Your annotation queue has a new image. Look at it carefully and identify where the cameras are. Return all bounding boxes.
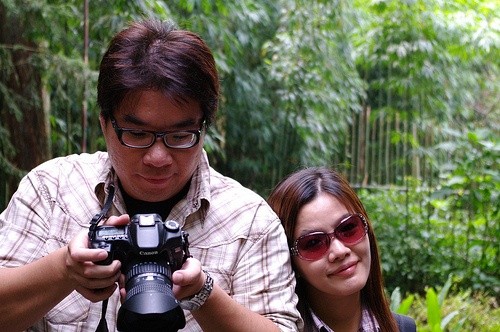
[91,214,190,332]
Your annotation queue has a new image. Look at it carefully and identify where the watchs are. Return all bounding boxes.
[174,269,214,313]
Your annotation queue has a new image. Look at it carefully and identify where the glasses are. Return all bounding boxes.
[108,112,206,149]
[291,213,368,262]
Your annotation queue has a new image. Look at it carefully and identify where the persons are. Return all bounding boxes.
[267,166,417,332]
[0,20,305,332]
